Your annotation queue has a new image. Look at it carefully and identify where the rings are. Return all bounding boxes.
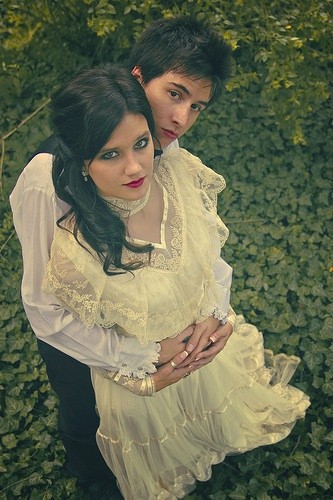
[169,360,178,369]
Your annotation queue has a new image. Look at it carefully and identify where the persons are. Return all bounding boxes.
[8,13,236,500]
[38,64,311,500]
